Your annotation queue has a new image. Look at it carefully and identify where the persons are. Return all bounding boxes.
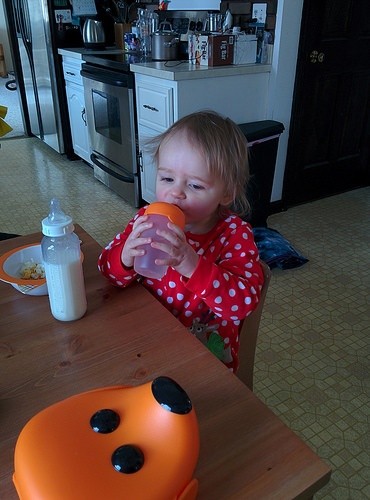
[98,110,264,374]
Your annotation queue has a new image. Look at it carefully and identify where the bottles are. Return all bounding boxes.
[135,201,186,277]
[41,199,87,321]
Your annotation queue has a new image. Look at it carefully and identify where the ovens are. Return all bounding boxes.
[81,64,144,208]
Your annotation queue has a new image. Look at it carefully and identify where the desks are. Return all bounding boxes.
[1,223,331,499]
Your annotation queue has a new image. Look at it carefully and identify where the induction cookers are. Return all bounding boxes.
[81,9,219,72]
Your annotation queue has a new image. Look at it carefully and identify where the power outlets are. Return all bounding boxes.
[252,3,266,23]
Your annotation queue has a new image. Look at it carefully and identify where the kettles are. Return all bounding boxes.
[80,16,106,50]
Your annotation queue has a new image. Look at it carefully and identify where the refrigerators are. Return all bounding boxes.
[10,0,118,160]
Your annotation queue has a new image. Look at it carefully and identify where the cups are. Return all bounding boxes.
[124,33,136,49]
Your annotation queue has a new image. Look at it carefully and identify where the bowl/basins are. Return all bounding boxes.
[0,242,83,295]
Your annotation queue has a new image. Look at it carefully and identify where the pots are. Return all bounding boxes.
[151,21,181,61]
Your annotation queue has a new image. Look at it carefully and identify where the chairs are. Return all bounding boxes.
[236,260,272,391]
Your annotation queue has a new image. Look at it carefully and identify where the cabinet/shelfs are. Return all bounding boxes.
[62,55,93,168]
[135,72,270,204]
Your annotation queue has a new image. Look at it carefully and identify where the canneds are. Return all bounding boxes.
[124,33,136,50]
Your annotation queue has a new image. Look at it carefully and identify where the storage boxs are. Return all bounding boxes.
[189,35,235,67]
